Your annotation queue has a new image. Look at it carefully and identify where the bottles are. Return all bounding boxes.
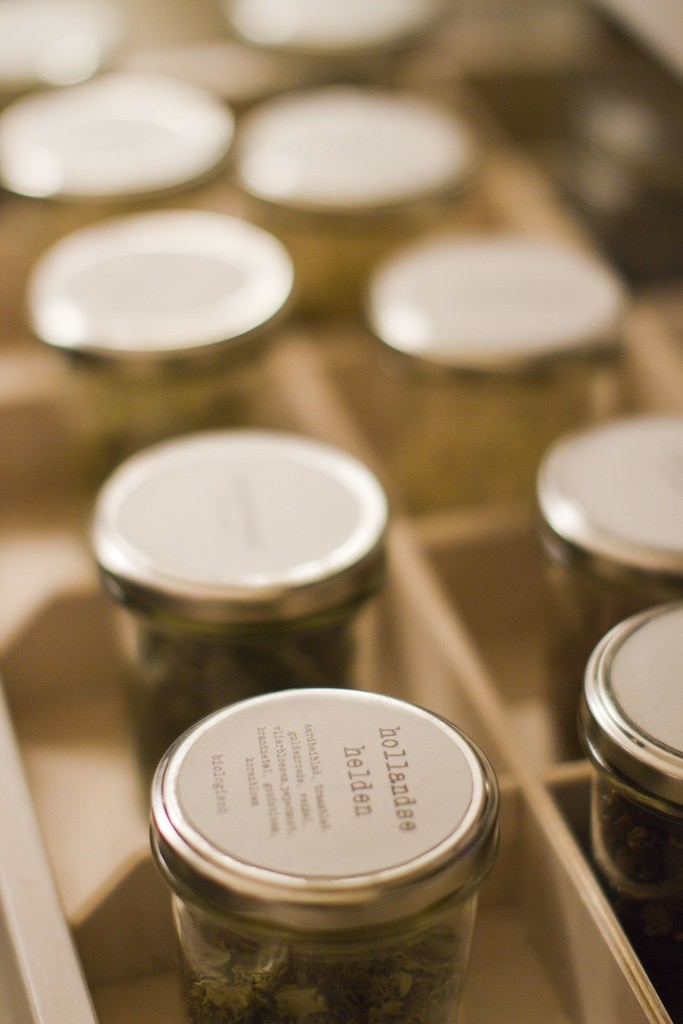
[1,0,683,1024]
